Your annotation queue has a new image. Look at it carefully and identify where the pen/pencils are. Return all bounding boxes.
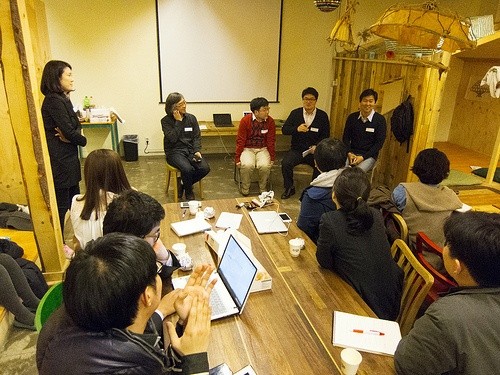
[182,209,186,217]
[353,328,385,336]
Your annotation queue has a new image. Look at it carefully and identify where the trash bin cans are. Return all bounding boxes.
[122,134,139,161]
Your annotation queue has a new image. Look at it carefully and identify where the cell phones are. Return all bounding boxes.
[352,158,356,162]
[279,212,292,223]
[194,155,202,162]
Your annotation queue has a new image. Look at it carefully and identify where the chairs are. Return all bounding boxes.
[367,147,383,190]
[388,213,460,338]
[162,131,205,203]
[234,154,273,194]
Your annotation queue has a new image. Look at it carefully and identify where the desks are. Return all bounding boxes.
[160,196,398,375]
[78,116,119,164]
[198,119,284,136]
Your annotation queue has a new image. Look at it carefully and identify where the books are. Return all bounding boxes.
[333,311,402,357]
[171,218,212,237]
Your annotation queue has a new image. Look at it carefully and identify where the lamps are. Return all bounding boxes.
[313,0,341,12]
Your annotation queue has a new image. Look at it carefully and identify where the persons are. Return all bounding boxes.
[342,89,387,173]
[394,210,500,375]
[0,253,40,331]
[391,148,463,271]
[281,87,330,199]
[161,92,210,201]
[296,137,348,246]
[35,231,217,375]
[71,149,138,250]
[102,191,183,334]
[236,97,275,196]
[40,60,87,262]
[316,167,405,321]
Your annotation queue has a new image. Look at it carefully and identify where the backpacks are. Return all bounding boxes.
[390,94,414,153]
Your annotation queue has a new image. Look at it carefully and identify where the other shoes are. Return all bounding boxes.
[63,244,75,259]
[177,177,185,199]
[242,188,249,196]
[21,301,41,312]
[260,189,267,194]
[14,316,37,330]
[186,187,195,201]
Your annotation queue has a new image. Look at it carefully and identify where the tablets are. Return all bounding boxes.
[180,202,201,208]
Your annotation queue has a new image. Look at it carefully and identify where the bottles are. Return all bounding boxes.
[83,96,95,109]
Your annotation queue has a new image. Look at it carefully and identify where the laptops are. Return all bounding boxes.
[213,114,234,127]
[249,210,288,235]
[168,233,258,323]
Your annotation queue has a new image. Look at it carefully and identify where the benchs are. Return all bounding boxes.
[0,204,42,347]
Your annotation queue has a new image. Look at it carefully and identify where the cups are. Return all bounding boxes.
[172,243,186,254]
[289,239,301,258]
[340,348,362,375]
[188,200,198,215]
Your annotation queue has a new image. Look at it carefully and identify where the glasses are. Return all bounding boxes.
[176,100,186,108]
[259,106,271,113]
[302,96,316,103]
[142,232,160,243]
[139,259,164,295]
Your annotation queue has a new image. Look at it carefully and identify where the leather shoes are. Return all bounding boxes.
[281,186,295,199]
